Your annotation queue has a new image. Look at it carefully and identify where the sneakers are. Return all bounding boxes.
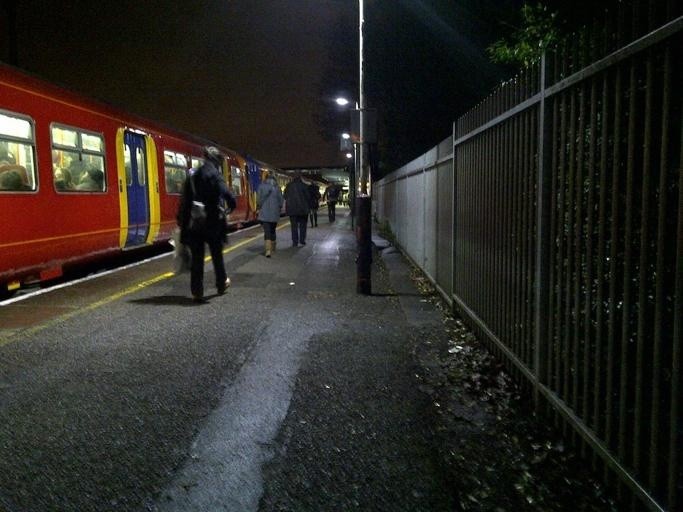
[218,277,230,294]
[193,290,205,302]
[292,240,306,247]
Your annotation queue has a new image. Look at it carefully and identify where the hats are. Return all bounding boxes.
[205,144,223,165]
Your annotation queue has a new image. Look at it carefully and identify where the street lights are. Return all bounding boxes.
[335,97,358,231]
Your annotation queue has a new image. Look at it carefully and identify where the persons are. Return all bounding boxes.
[283,176,311,246]
[307,181,321,227]
[256,174,283,258]
[0,143,104,192]
[176,145,236,303]
[323,183,338,223]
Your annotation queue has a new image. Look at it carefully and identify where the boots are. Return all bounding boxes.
[310,213,317,228]
[264,240,271,257]
[270,241,276,253]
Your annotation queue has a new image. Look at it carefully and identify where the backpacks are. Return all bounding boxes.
[329,187,337,198]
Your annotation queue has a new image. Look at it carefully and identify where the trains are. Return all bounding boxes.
[0,63,328,301]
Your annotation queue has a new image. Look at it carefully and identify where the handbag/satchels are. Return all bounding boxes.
[190,202,225,233]
